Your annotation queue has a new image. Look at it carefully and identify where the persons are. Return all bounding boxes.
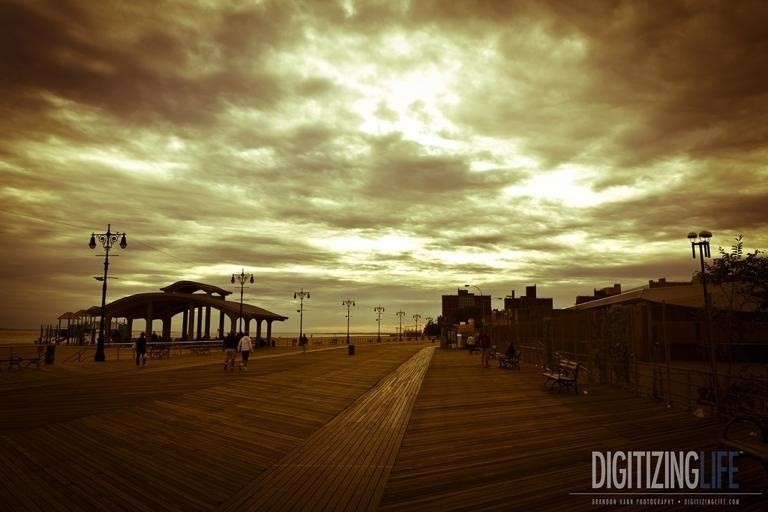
[504,342,517,368]
[151,331,158,342]
[478,328,493,369]
[135,332,147,367]
[302,333,308,344]
[223,328,253,372]
[466,334,476,355]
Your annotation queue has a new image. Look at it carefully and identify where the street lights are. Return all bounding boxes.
[341,299,433,343]
[88,223,127,362]
[464,284,486,325]
[293,287,310,346]
[687,230,723,423]
[231,268,254,332]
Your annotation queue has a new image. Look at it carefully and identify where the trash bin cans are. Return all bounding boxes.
[45,346,55,363]
[457,336,463,348]
[255,340,260,348]
[432,339,435,343]
[272,341,275,347]
[440,336,448,349]
[349,346,355,356]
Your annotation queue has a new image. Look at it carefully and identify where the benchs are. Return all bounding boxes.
[191,346,211,355]
[719,417,767,474]
[541,359,582,394]
[8,355,40,371]
[149,347,169,359]
[503,351,521,369]
